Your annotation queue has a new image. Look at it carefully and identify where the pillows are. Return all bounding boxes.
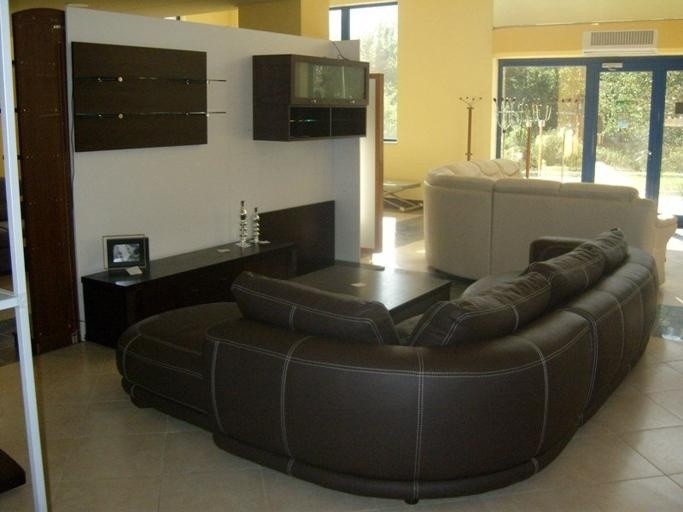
[408,272,552,346]
[230,269,402,346]
[573,227,630,271]
[519,247,606,312]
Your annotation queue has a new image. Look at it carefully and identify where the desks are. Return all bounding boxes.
[383,179,423,213]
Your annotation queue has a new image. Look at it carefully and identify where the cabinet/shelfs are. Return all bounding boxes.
[252,54,370,141]
[81,239,296,350]
[71,41,227,153]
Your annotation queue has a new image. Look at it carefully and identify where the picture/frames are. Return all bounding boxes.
[103,234,150,276]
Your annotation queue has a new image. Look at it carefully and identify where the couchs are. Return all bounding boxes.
[117,235,659,505]
[421,159,678,286]
